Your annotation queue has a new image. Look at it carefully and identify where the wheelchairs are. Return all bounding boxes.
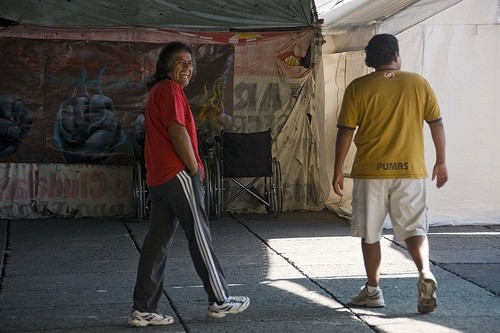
[214,128,283,221]
[133,133,213,223]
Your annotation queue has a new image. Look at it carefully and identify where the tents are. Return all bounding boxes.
[0,0,500,230]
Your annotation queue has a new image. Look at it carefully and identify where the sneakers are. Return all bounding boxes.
[127,310,175,327]
[351,282,386,308]
[417,272,438,313]
[207,295,250,318]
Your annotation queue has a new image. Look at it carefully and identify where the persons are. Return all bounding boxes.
[332,32,449,313]
[0,95,33,162]
[128,42,251,326]
[54,95,121,165]
